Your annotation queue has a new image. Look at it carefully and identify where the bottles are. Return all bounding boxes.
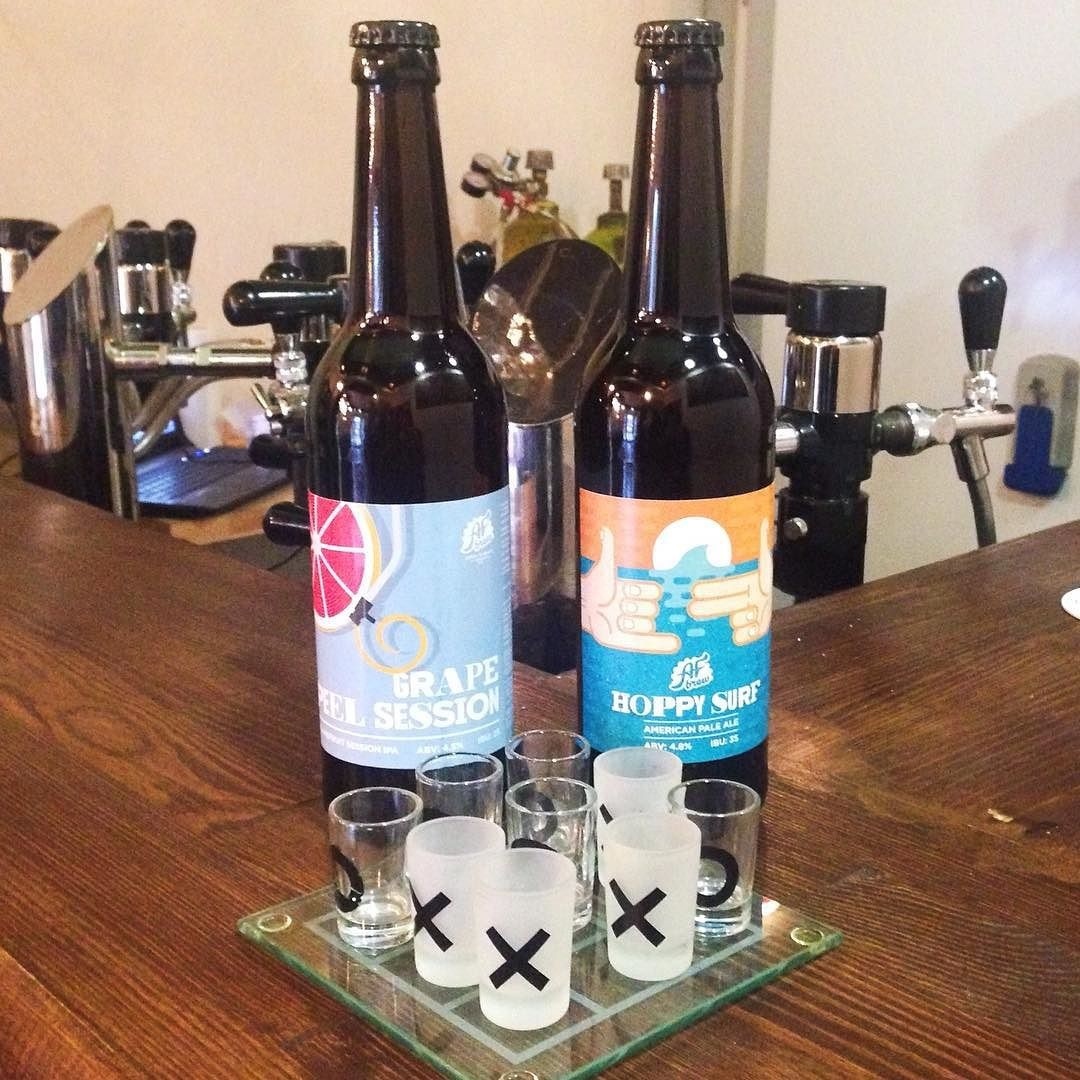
[298,18,517,812]
[568,14,781,827]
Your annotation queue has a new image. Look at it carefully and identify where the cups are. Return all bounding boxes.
[327,725,760,1030]
[504,413,573,606]
